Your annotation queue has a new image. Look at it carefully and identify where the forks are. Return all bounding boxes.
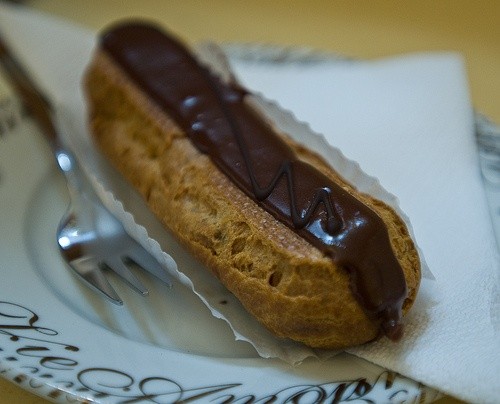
[0,30,175,306]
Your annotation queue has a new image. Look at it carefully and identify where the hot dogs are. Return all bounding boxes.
[80,16,421,350]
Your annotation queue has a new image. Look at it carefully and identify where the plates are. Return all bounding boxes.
[0,45,498,404]
